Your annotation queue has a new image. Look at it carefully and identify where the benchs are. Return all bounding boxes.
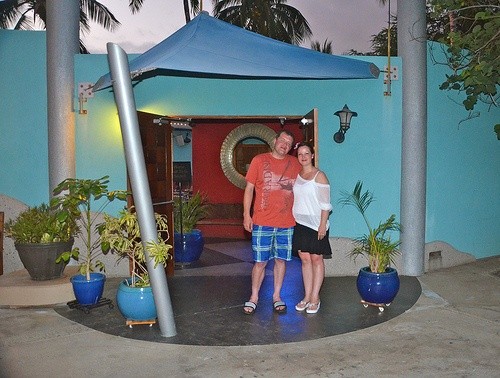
[193,203,253,239]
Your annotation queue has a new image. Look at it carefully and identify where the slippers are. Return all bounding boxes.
[273,301,286,312]
[306,301,320,314]
[295,301,310,311]
[243,302,256,314]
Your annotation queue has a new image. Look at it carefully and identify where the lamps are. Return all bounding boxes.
[334,104,358,142]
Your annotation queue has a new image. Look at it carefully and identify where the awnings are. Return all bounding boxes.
[91,10,381,93]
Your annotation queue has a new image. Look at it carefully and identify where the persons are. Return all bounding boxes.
[243,130,302,315]
[292,143,332,314]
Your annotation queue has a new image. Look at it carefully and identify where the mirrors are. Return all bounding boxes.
[220,123,278,190]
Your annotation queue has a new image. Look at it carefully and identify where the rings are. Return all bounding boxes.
[322,234,324,236]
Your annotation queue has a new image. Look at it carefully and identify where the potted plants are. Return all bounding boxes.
[173,190,211,263]
[339,179,404,303]
[51,176,132,305]
[103,205,173,320]
[2,202,81,281]
